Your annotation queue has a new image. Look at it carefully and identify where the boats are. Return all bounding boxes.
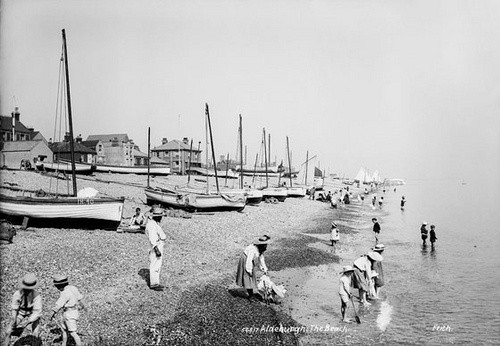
[96,164,174,177]
[36,154,98,176]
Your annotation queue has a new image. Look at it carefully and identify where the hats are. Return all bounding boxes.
[339,265,357,274]
[254,234,272,244]
[369,252,383,261]
[373,244,386,252]
[332,222,337,227]
[152,207,164,217]
[18,275,39,289]
[52,275,69,284]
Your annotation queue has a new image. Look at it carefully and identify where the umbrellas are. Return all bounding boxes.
[75,187,99,201]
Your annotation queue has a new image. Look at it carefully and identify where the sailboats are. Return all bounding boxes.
[1,28,126,231]
[353,164,386,187]
[224,126,288,203]
[185,141,240,180]
[251,136,306,199]
[285,167,301,178]
[235,132,286,178]
[184,113,263,205]
[143,102,248,215]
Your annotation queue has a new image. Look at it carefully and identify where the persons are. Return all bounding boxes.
[140,204,159,228]
[49,276,88,346]
[5,273,42,346]
[360,244,386,300]
[128,208,144,226]
[145,208,165,291]
[421,221,428,248]
[339,265,358,322]
[305,185,350,209]
[330,222,340,246]
[365,252,383,300]
[372,218,380,244]
[379,197,384,208]
[351,257,371,307]
[401,197,406,210]
[236,235,273,302]
[372,197,377,206]
[430,225,437,252]
